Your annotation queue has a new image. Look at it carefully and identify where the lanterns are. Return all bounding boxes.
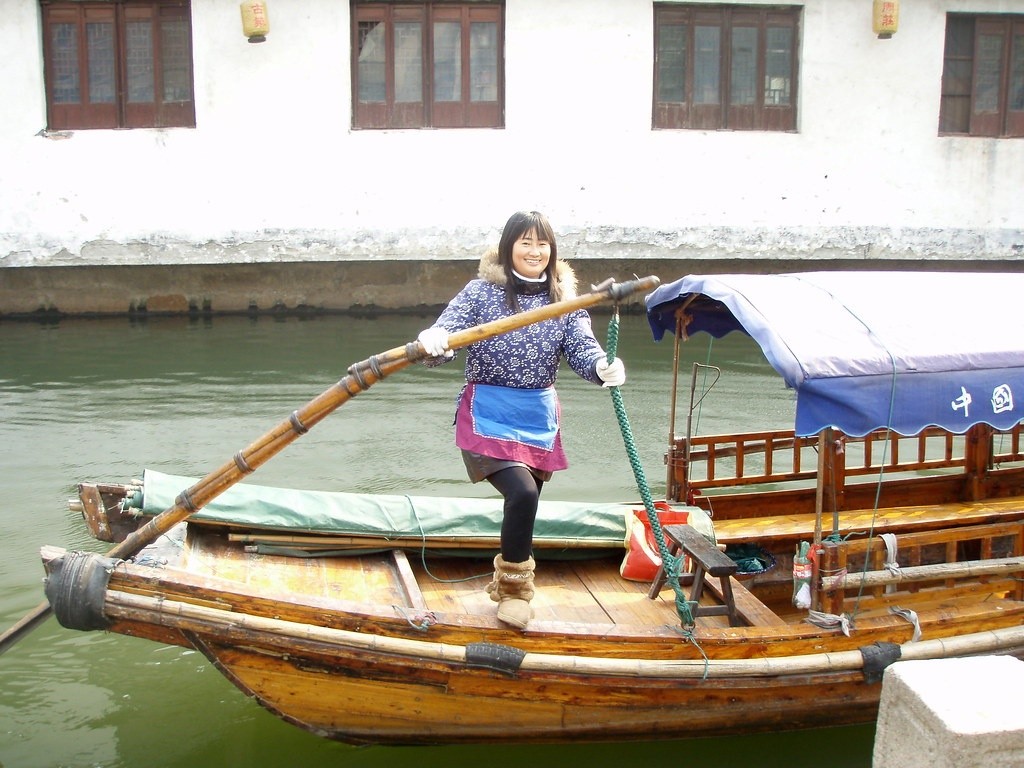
[240,0,270,43]
[873,0,900,39]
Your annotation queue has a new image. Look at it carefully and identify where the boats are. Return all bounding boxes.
[33,273,1024,748]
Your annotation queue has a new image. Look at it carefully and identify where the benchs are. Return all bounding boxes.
[712,496,1024,544]
[648,524,737,626]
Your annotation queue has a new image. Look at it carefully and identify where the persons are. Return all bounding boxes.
[418,211,626,628]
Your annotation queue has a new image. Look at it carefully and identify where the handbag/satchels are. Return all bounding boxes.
[619,501,693,581]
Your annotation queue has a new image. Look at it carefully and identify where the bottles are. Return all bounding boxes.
[791,555,813,609]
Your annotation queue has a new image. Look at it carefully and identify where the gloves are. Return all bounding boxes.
[418,327,456,359]
[595,357,625,387]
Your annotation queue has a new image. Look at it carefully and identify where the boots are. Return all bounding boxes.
[484,554,537,630]
